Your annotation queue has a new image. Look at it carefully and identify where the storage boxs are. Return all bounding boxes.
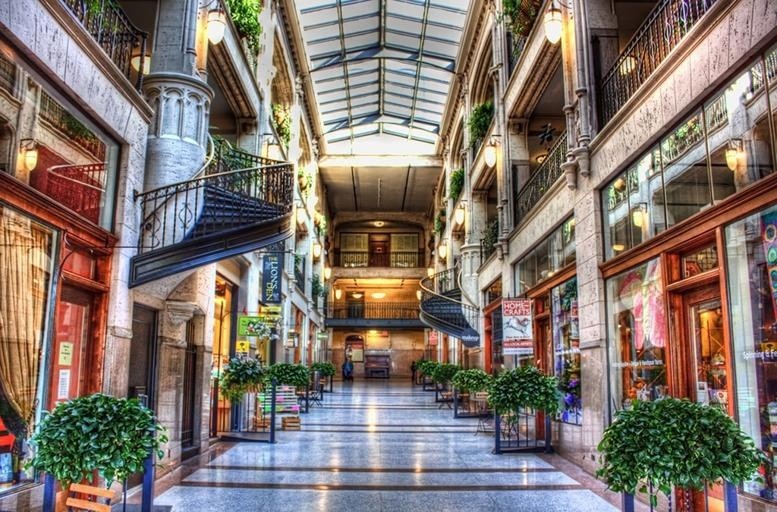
[254,418,272,433]
[281,416,301,431]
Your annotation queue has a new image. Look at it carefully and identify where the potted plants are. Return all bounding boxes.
[212,353,273,406]
[297,167,314,192]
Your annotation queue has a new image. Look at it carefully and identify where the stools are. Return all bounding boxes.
[437,391,499,437]
[295,389,321,414]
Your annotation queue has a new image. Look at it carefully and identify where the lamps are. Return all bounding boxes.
[544,0,572,46]
[201,1,227,46]
[633,202,647,228]
[294,206,344,302]
[411,134,503,302]
[19,137,40,172]
[724,137,744,171]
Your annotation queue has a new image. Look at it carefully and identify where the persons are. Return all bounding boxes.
[342,356,354,383]
[411,361,416,383]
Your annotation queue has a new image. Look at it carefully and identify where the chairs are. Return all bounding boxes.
[65,482,116,512]
